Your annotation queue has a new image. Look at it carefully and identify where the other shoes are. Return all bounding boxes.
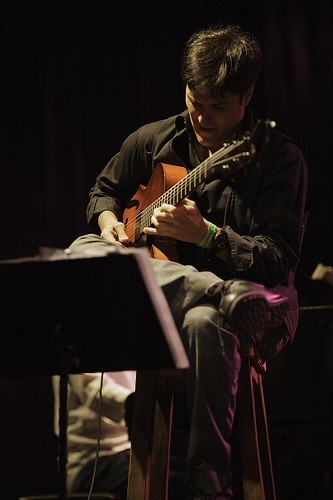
[215,279,288,331]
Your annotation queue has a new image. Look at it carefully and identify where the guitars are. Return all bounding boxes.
[118,119,277,264]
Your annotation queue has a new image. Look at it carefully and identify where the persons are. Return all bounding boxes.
[38,25,307,500]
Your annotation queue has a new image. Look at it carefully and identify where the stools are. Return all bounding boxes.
[127,343,277,500]
